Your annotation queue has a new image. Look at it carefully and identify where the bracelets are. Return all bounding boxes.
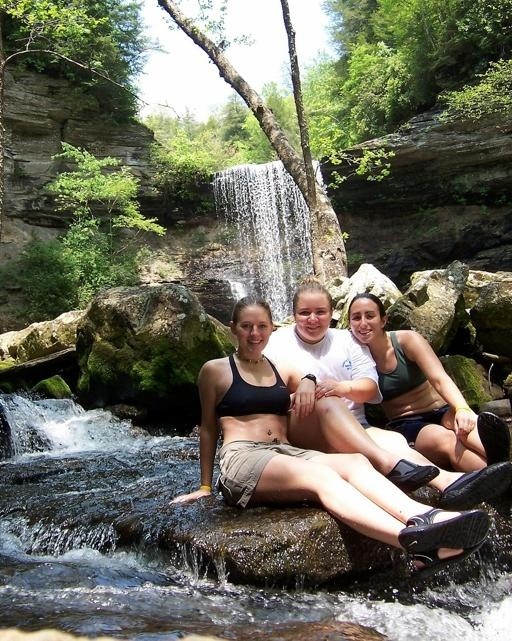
[453,403,473,415]
[199,485,213,493]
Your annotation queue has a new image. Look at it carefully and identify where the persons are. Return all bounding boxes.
[167,294,492,580]
[348,293,512,521]
[258,277,512,509]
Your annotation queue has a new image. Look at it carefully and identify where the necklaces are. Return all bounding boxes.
[235,349,267,365]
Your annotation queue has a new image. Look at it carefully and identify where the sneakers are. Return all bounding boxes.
[441,461,512,509]
[384,460,439,494]
[478,412,510,466]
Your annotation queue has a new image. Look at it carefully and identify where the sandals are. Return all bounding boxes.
[408,541,486,580]
[398,509,490,552]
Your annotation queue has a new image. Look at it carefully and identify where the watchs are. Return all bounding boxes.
[300,374,317,387]
